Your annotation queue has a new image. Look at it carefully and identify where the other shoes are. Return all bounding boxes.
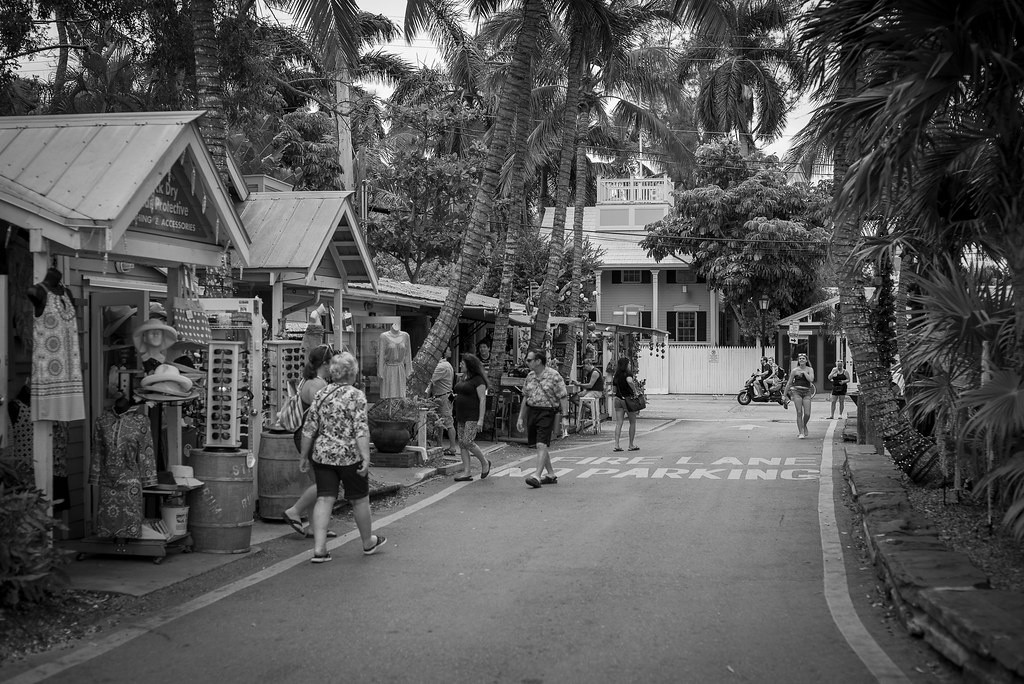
[481,461,491,479]
[799,427,808,438]
[455,475,473,481]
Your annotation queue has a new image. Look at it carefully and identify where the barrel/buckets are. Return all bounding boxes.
[161,506,189,535]
[189,448,254,554]
[258,431,316,519]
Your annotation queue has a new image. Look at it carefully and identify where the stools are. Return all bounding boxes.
[576,397,600,436]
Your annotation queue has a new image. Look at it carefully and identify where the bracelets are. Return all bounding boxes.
[562,415,568,419]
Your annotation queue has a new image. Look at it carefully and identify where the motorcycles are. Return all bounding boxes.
[737,368,791,405]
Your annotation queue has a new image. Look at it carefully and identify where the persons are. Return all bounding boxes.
[826,362,850,419]
[475,338,491,374]
[442,346,451,361]
[8,375,72,511]
[763,357,782,395]
[612,358,640,451]
[281,345,337,537]
[784,354,814,438]
[300,304,326,350]
[517,348,569,488]
[376,322,412,399]
[297,351,387,562]
[87,397,159,538]
[570,358,604,417]
[431,356,455,456]
[454,353,492,482]
[754,356,772,398]
[141,329,165,376]
[26,268,86,421]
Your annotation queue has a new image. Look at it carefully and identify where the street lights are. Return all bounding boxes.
[759,291,771,373]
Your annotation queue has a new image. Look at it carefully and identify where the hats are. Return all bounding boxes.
[171,464,205,487]
[103,306,138,338]
[133,318,177,353]
[133,356,205,401]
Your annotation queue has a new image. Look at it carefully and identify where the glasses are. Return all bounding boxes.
[318,345,329,361]
[798,353,806,357]
[211,347,305,440]
[527,358,536,362]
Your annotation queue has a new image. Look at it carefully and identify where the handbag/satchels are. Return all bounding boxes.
[173,264,212,348]
[625,389,646,412]
[552,412,561,436]
[783,398,788,409]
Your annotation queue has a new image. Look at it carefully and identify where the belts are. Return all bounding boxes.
[435,390,453,398]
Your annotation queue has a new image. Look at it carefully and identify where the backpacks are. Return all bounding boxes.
[778,367,785,379]
[280,375,326,433]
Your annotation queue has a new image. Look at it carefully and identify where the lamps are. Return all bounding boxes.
[115,261,134,273]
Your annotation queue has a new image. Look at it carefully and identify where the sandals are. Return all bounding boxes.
[526,477,541,488]
[541,476,557,483]
[443,448,456,455]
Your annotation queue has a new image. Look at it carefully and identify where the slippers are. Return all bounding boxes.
[311,552,332,562]
[613,447,624,451]
[630,445,639,450]
[363,535,388,555]
[305,530,335,538]
[281,510,305,536]
